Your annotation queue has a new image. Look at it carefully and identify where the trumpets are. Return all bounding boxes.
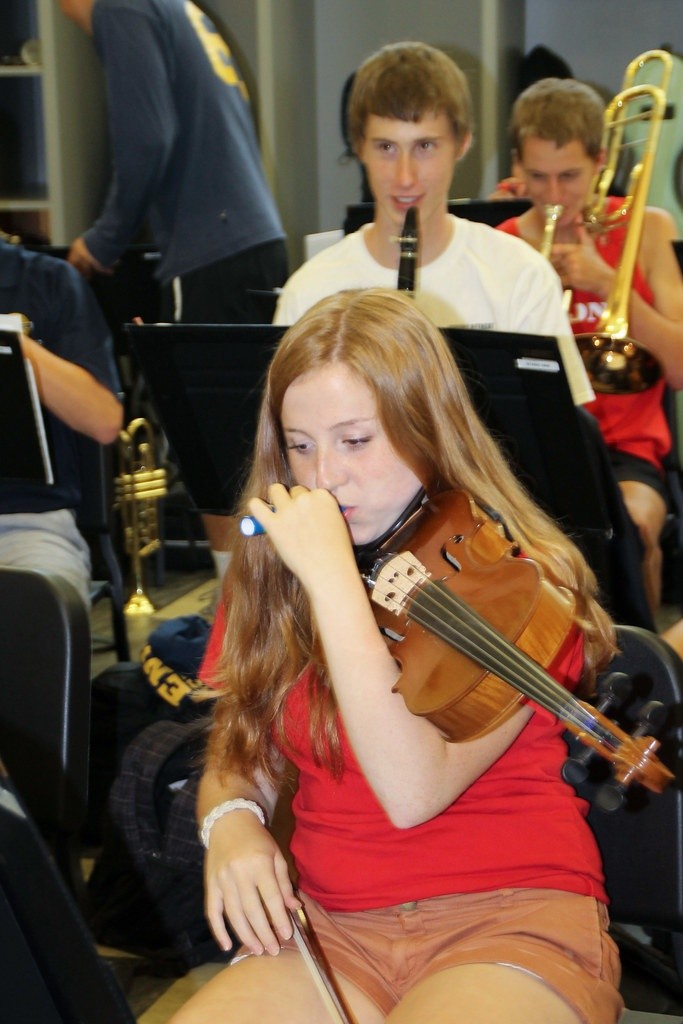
[115,418,167,616]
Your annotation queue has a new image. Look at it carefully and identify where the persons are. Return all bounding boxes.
[0,241,124,612]
[274,39,683,674]
[56,0,288,583]
[166,288,622,1024]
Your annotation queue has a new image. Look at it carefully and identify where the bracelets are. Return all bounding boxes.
[200,797,265,849]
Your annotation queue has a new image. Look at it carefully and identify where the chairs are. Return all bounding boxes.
[0,434,131,1024]
[561,625,683,1024]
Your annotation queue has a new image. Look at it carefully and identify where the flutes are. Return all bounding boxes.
[393,208,419,292]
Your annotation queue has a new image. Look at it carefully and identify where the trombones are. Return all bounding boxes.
[540,50,675,395]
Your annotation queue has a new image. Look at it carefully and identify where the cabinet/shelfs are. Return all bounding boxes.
[1,0,523,279]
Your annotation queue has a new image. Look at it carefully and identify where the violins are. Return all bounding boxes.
[362,485,676,810]
